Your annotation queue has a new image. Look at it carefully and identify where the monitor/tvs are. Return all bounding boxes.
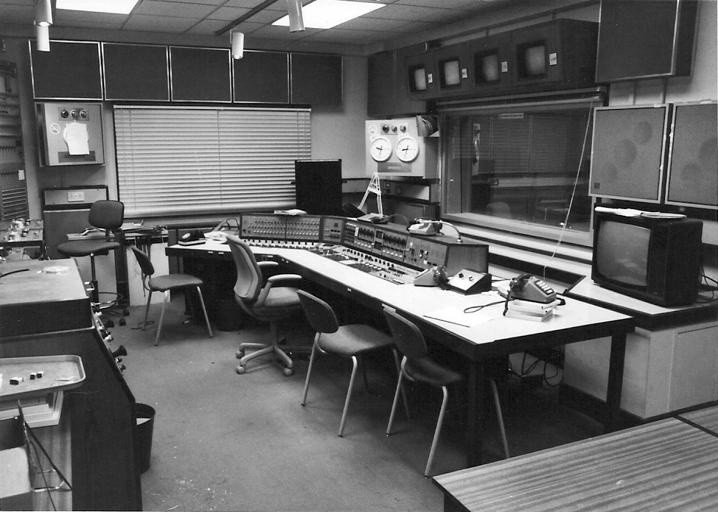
[591,210,703,308]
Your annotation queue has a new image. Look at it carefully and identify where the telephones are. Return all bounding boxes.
[509,272,557,303]
[413,264,450,287]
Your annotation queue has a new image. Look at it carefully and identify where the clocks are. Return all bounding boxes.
[368,137,392,162]
[394,137,420,163]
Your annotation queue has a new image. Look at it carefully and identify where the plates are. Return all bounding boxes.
[44,265,69,273]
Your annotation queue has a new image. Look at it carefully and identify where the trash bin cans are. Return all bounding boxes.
[136,403,156,473]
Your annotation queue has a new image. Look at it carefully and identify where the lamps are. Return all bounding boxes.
[36,0,54,27]
[231,32,244,61]
[287,0,304,33]
[36,21,51,53]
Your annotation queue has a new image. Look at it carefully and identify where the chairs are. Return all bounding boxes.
[131,246,214,346]
[296,288,413,437]
[56,199,130,329]
[225,234,316,377]
[379,304,510,477]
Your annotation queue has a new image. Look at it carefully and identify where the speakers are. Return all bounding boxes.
[588,104,667,205]
[664,100,716,210]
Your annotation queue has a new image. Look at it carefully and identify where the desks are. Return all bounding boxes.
[165,240,302,315]
[277,250,633,468]
[678,406,716,436]
[431,417,718,512]
[66,232,167,307]
